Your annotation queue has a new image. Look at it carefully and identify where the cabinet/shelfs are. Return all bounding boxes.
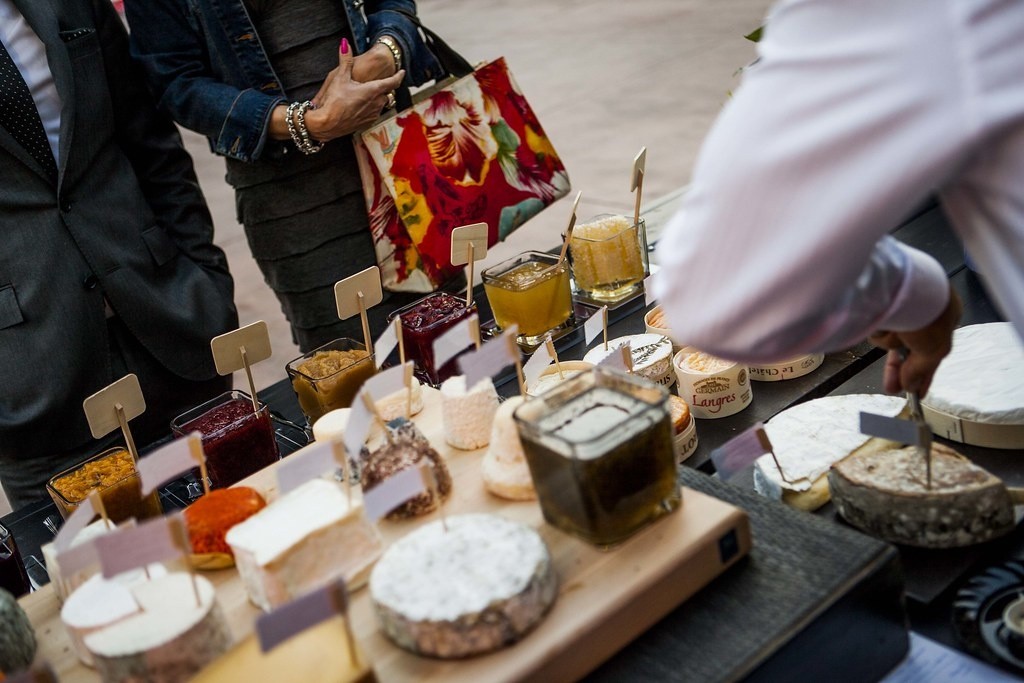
[0,188,1024,683]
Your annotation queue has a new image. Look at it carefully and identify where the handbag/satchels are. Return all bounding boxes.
[353,7,571,293]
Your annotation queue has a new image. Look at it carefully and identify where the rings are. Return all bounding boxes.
[386,92,397,109]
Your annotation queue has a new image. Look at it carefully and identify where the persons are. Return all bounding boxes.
[650,1,1024,395]
[0,0,238,511]
[122,0,452,365]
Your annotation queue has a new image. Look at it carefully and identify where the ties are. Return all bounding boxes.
[0,39,58,178]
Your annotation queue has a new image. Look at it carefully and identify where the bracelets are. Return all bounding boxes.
[284,101,325,156]
[377,37,401,71]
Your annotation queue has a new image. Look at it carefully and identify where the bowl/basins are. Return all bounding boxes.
[673,346,754,419]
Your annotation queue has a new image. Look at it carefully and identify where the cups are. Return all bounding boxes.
[45,446,142,527]
[386,291,479,390]
[285,337,376,428]
[480,250,576,345]
[513,364,682,550]
[169,390,280,493]
[561,215,651,304]
